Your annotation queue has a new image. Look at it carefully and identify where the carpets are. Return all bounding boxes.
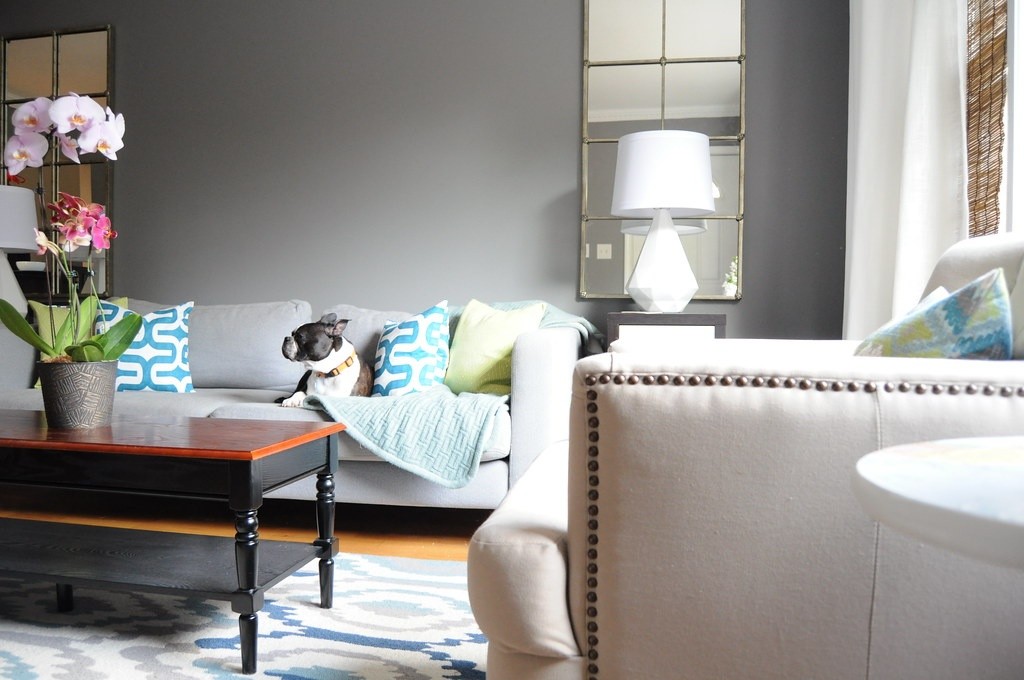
[1,551,489,680]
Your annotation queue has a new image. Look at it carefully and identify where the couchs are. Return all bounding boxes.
[466,231,1024,680]
[0,299,583,510]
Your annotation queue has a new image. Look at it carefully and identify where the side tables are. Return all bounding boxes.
[607,312,727,350]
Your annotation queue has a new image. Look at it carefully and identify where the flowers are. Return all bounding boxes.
[722,256,738,296]
[3,95,143,364]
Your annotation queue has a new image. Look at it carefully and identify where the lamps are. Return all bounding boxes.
[611,130,714,313]
[0,185,40,319]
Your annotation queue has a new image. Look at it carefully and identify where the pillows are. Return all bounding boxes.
[371,299,451,397]
[28,301,129,387]
[94,299,195,392]
[854,266,1013,360]
[445,299,547,396]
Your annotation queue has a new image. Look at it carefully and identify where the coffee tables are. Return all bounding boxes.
[0,409,347,673]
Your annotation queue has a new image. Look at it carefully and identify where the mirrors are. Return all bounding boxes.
[578,0,744,302]
[0,22,118,295]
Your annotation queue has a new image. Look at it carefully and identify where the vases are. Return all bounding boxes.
[36,361,117,430]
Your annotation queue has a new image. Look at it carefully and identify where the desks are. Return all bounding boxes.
[857,433,1023,571]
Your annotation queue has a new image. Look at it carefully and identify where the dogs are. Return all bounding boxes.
[273,313,374,408]
